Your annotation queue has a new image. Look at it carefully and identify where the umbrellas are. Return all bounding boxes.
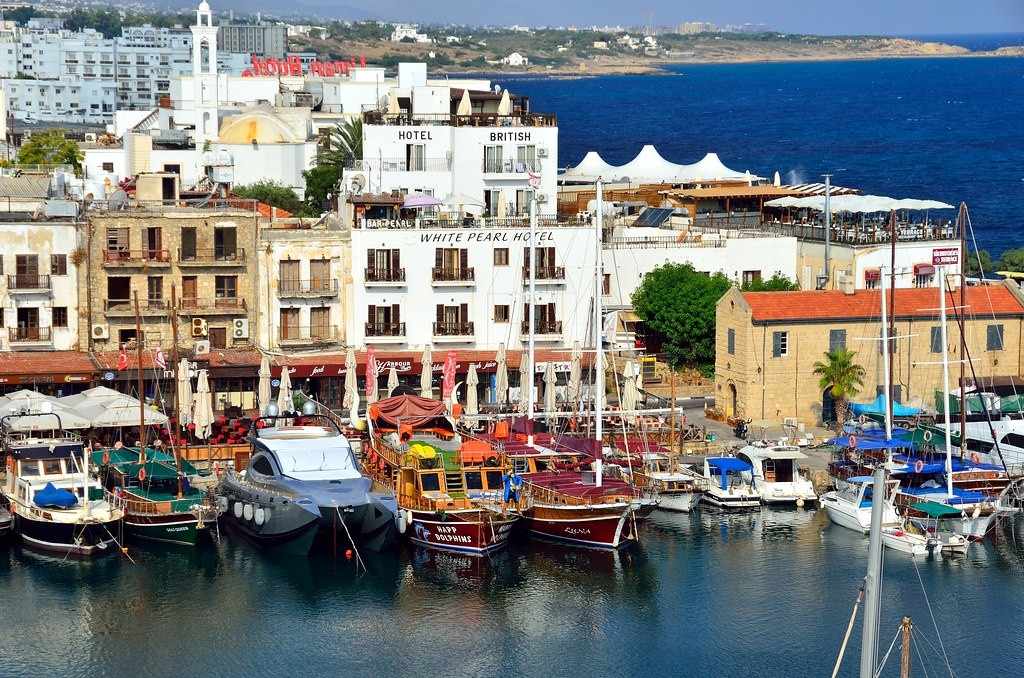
[542,362,556,433]
[277,366,294,427]
[178,358,193,446]
[193,370,215,445]
[495,342,508,410]
[594,351,636,432]
[696,166,780,187]
[568,340,583,403]
[403,191,485,218]
[421,345,478,433]
[258,358,271,424]
[764,193,955,234]
[342,349,400,412]
[520,352,529,412]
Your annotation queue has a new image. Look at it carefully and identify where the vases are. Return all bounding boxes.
[707,414,724,422]
[727,421,736,427]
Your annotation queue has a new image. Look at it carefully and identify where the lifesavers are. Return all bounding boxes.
[368,448,372,458]
[373,453,377,462]
[139,468,146,481]
[364,444,368,452]
[102,452,109,464]
[379,459,384,469]
[6,455,12,466]
[10,502,15,515]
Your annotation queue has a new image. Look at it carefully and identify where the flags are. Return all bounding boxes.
[117,348,128,371]
[156,344,166,371]
[528,173,541,188]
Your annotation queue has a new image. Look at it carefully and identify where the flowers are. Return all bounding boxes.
[704,409,724,417]
[726,416,745,424]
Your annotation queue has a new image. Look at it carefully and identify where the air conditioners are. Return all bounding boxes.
[194,340,210,354]
[233,319,249,338]
[783,418,797,429]
[192,318,207,336]
[537,147,549,157]
[537,193,548,202]
[91,323,109,339]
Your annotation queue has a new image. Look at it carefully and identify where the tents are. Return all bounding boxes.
[849,394,924,418]
[573,145,761,184]
[0,386,177,459]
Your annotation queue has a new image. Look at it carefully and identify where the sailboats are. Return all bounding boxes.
[817,201,1024,556]
[472,177,662,549]
[87,285,222,547]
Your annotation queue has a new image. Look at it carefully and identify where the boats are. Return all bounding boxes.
[0,378,127,562]
[641,365,818,512]
[216,413,398,557]
[358,392,534,557]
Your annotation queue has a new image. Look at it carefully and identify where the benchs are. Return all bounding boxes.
[374,426,455,441]
